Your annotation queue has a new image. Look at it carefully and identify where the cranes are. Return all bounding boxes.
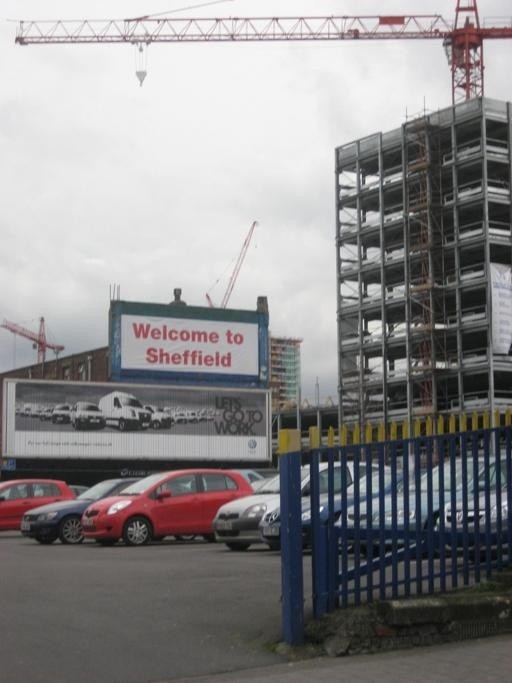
[204,220,258,312]
[8,0,512,105]
[0,313,65,363]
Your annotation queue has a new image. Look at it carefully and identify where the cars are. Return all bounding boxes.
[17,390,217,431]
